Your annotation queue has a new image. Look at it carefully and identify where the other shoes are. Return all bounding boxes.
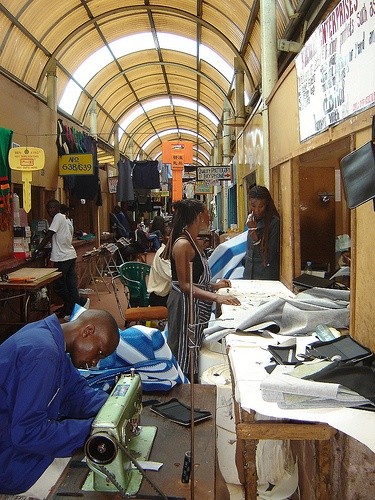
[80,297,90,309]
[57,310,72,318]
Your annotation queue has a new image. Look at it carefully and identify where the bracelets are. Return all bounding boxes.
[34,249,39,255]
[248,228,258,237]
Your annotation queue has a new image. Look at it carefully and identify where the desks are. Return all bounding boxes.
[216,279,336,500]
[0,268,62,330]
[46,384,216,500]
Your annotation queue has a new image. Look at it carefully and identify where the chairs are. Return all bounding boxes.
[111,274,168,329]
[120,261,151,320]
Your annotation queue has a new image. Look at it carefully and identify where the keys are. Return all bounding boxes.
[161,139,193,203]
[8,146,45,214]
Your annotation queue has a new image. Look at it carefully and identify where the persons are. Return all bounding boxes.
[27,200,88,320]
[244,185,281,280]
[166,197,241,385]
[113,205,172,307]
[0,309,140,500]
